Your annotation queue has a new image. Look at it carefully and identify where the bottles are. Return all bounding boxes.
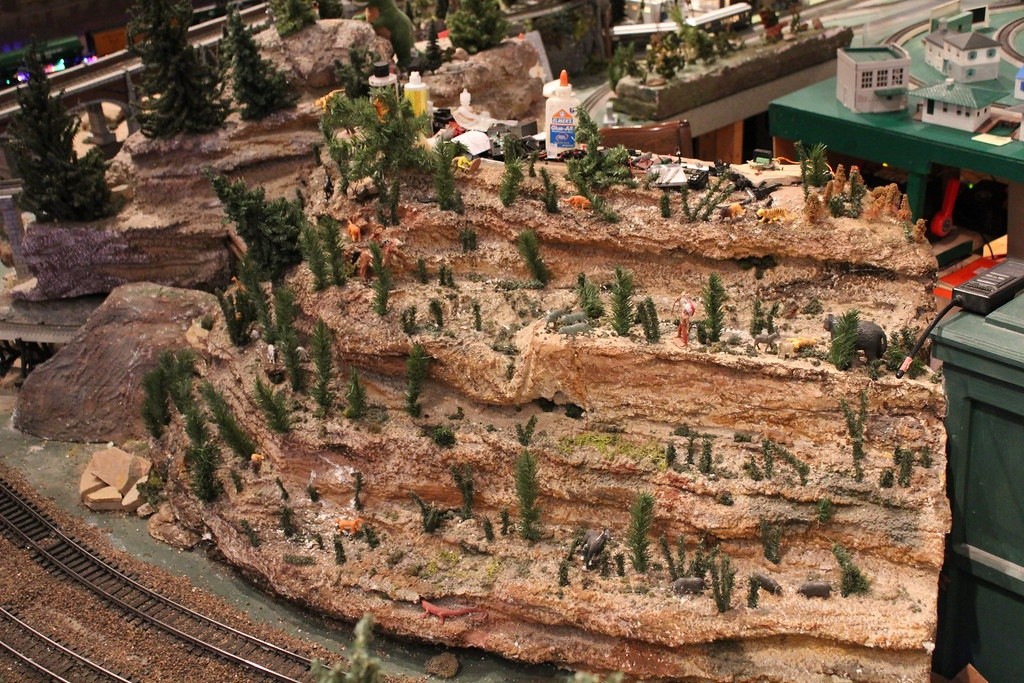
[456,88,476,125]
[546,72,581,159]
[367,61,399,124]
[404,70,429,117]
[432,109,455,135]
